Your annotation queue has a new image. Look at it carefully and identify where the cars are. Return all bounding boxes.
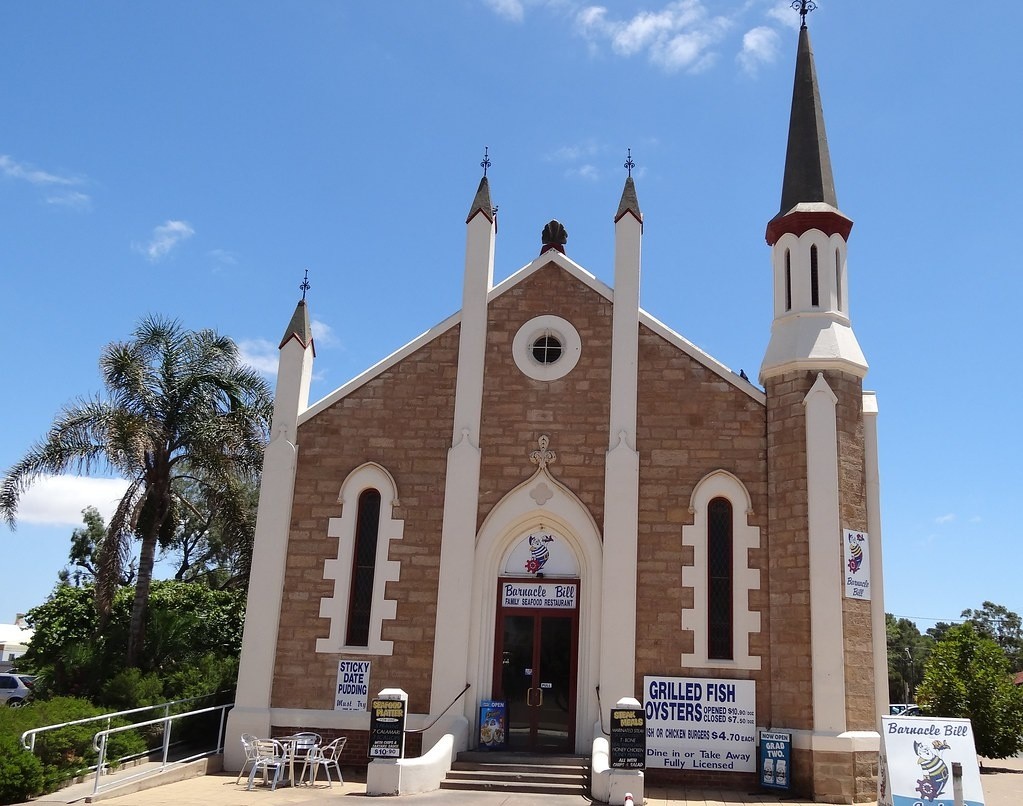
[891,704,934,718]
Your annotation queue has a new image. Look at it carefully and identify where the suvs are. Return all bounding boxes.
[0,671,40,708]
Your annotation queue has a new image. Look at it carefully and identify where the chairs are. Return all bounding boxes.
[298,736,347,790]
[236,732,284,787]
[282,732,323,788]
[245,739,290,792]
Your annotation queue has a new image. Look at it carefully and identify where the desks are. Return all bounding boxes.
[272,737,319,788]
